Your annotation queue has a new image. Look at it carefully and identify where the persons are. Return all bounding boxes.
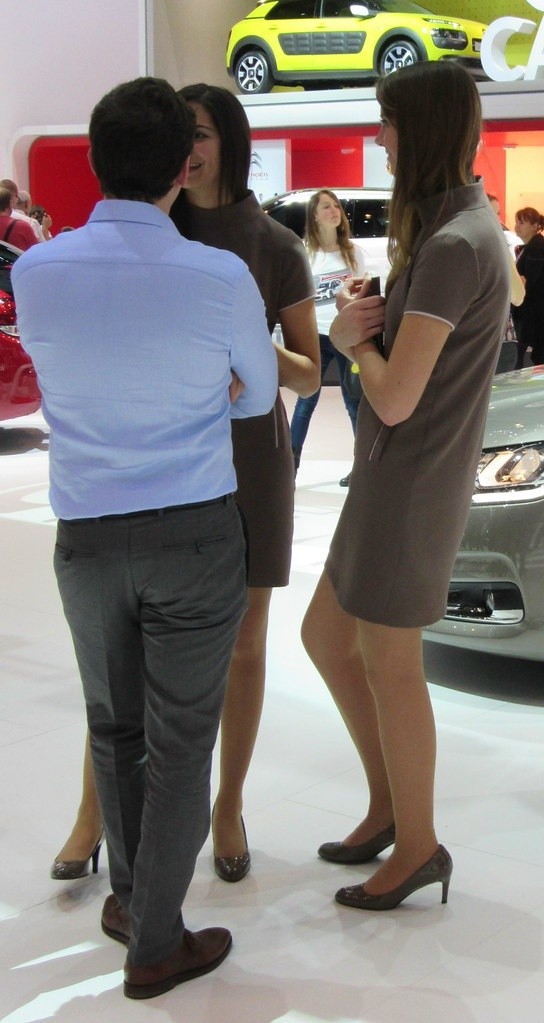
[290,189,366,492]
[486,193,544,375]
[1,76,322,997]
[300,60,512,910]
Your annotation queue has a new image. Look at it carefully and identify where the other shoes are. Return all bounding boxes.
[339,471,352,486]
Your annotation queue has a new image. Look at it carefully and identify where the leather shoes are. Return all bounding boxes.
[101,893,132,945]
[123,927,232,999]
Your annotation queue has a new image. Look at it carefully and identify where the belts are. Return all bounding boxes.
[99,491,235,520]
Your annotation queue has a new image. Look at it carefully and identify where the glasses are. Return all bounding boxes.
[15,194,20,205]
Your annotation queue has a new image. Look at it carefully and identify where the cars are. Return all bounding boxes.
[417,364,544,660]
[227,0,492,94]
[264,186,522,299]
[0,241,42,423]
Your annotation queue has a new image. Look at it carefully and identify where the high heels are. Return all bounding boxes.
[317,822,395,863]
[50,828,106,879]
[334,844,453,911]
[212,803,251,882]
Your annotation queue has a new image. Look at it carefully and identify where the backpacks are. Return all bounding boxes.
[0,240,25,298]
